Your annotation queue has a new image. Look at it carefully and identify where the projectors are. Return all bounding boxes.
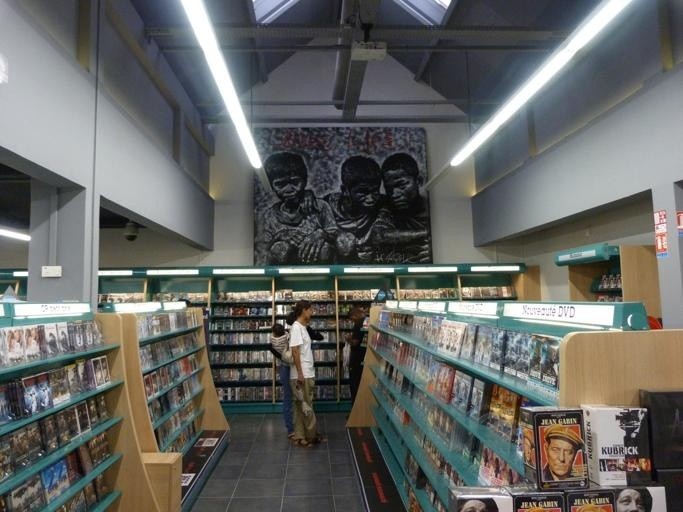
[351,39,387,62]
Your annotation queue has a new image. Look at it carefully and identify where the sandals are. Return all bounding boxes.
[293,437,312,448]
[288,431,294,439]
[308,436,329,447]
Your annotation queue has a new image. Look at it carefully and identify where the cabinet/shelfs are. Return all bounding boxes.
[554,244,661,319]
[208,275,274,413]
[273,275,337,414]
[345,302,683,512]
[102,301,231,512]
[144,278,209,328]
[97,279,146,304]
[337,273,399,413]
[460,274,523,301]
[0,279,21,294]
[0,302,161,512]
[398,274,462,300]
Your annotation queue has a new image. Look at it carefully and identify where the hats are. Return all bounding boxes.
[522,428,535,447]
[545,424,587,451]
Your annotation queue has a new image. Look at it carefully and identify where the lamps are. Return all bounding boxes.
[417,0,636,199]
[0,228,32,243]
[179,0,272,194]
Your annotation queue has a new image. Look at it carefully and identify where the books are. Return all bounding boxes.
[314,366,337,379]
[94,473,113,500]
[148,371,204,425]
[341,366,349,379]
[88,397,99,423]
[462,286,515,298]
[26,421,44,461]
[164,407,206,455]
[484,385,520,441]
[505,485,565,512]
[0,371,54,427]
[313,349,336,362]
[66,452,79,481]
[96,395,113,420]
[46,368,69,402]
[340,303,353,312]
[135,309,199,342]
[362,334,368,345]
[314,385,336,400]
[406,455,426,490]
[362,317,370,328]
[340,331,353,342]
[517,397,546,458]
[66,489,88,512]
[225,291,272,302]
[68,319,84,352]
[400,288,458,299]
[55,504,67,512]
[138,330,203,373]
[640,387,683,468]
[378,358,461,455]
[341,385,351,399]
[312,303,336,314]
[210,317,272,331]
[489,328,507,371]
[39,466,62,504]
[407,489,424,512]
[276,385,285,401]
[617,488,653,512]
[374,331,472,418]
[154,394,201,450]
[5,484,31,512]
[74,358,90,391]
[540,336,563,397]
[84,481,98,510]
[84,320,104,348]
[209,332,272,345]
[309,318,336,329]
[276,304,294,315]
[82,323,93,349]
[564,490,616,512]
[10,427,29,468]
[375,378,466,487]
[74,400,92,436]
[474,324,493,367]
[378,309,476,358]
[276,289,336,300]
[55,411,71,449]
[468,378,493,424]
[77,444,93,475]
[26,475,47,512]
[311,331,336,343]
[215,386,273,401]
[142,352,208,399]
[0,435,16,483]
[461,434,484,467]
[526,333,554,389]
[654,468,683,512]
[599,273,622,287]
[211,367,273,382]
[88,433,112,464]
[338,289,398,301]
[209,350,273,364]
[340,317,354,329]
[444,460,466,487]
[152,292,208,303]
[404,454,447,512]
[581,403,653,485]
[597,296,622,302]
[66,405,81,440]
[63,363,78,394]
[212,305,272,317]
[39,415,58,454]
[503,328,521,376]
[423,435,444,473]
[123,293,143,303]
[516,332,532,381]
[54,459,70,494]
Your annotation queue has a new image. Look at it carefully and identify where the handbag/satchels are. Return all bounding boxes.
[281,323,304,365]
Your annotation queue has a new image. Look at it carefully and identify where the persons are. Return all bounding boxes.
[48,333,58,353]
[508,469,514,486]
[279,312,299,441]
[93,359,103,385]
[262,152,338,265]
[25,328,40,355]
[482,448,489,467]
[60,330,68,351]
[289,300,328,449]
[101,358,110,382]
[372,152,431,264]
[102,299,106,302]
[14,329,24,348]
[543,424,585,482]
[6,329,24,360]
[323,155,386,265]
[344,308,365,405]
[459,497,499,512]
[116,298,122,302]
[109,298,114,303]
[31,328,39,342]
[493,458,500,478]
[217,292,225,301]
[271,323,289,355]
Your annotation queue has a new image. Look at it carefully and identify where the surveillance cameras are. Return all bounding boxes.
[124,222,138,242]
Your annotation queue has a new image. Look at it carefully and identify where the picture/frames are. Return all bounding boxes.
[253,126,433,266]
